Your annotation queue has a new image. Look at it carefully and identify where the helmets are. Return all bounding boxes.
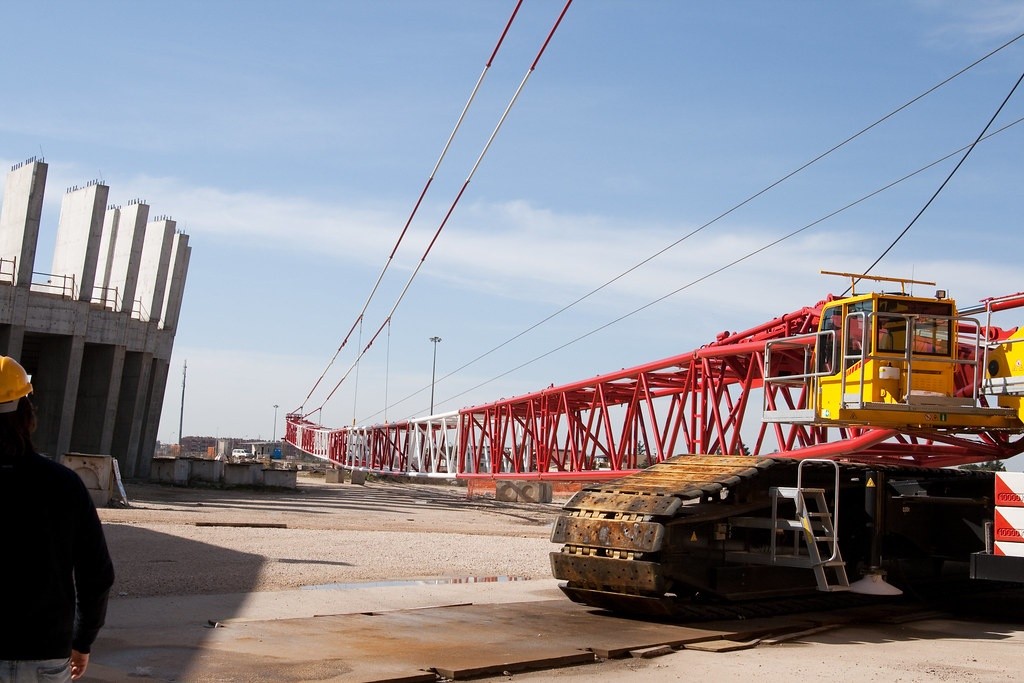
[0,355,33,412]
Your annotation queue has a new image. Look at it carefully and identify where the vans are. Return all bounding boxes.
[593,455,609,467]
[232,449,253,458]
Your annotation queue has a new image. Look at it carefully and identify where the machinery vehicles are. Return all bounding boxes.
[550,270,1024,626]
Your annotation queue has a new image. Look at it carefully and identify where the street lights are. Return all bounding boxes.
[273,405,278,443]
[429,336,443,415]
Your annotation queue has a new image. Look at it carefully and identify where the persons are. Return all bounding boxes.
[0,354,115,683]
[869,319,891,350]
